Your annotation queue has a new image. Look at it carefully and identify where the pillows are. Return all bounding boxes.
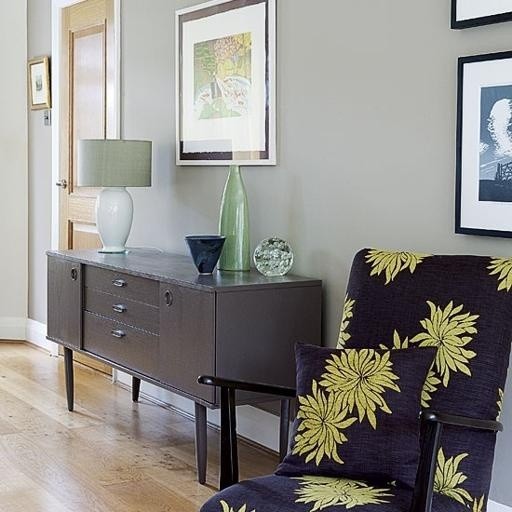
[273,341,439,489]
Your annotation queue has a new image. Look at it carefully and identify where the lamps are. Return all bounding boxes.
[77,139,152,254]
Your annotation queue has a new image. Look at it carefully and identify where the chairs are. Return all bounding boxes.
[196,249,512,512]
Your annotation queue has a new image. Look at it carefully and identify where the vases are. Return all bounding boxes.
[219,163,250,272]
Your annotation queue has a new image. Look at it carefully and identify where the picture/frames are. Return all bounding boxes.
[174,0,277,166]
[450,0,512,30]
[454,50,512,240]
[28,56,50,111]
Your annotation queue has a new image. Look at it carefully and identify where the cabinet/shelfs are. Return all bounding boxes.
[47,248,323,485]
